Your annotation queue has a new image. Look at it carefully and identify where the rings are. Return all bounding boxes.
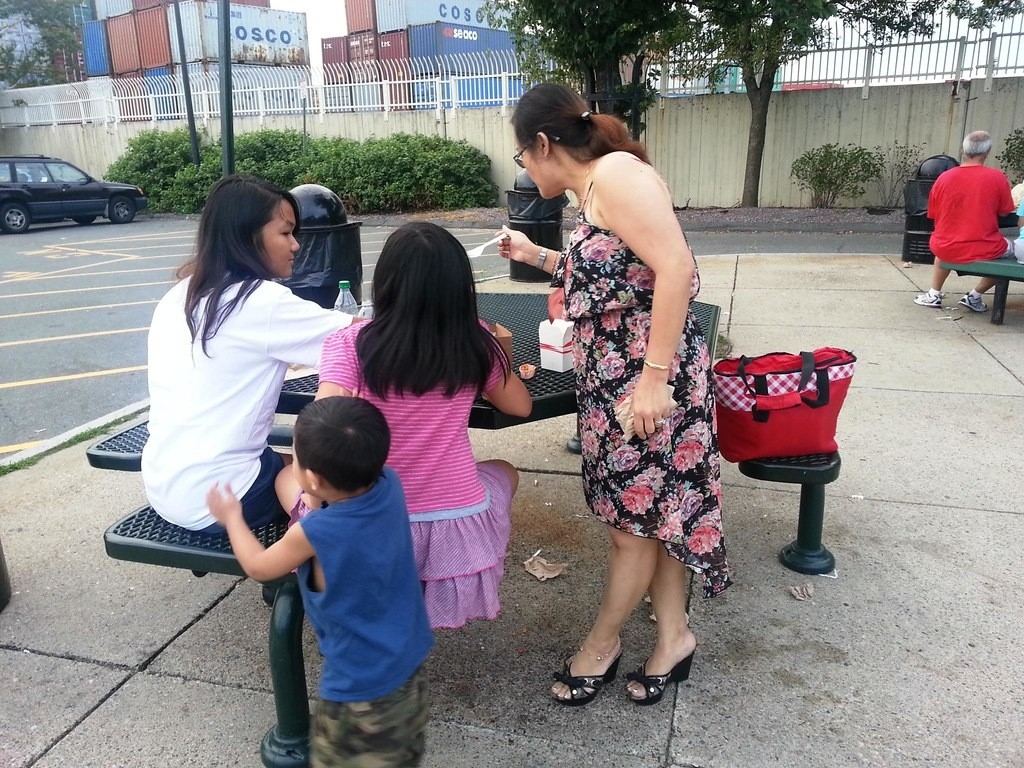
[655,419,667,425]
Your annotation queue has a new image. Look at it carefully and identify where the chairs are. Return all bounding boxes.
[18,174,29,182]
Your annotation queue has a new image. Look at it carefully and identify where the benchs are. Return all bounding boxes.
[85,419,151,478]
[104,510,316,768]
[939,257,1024,325]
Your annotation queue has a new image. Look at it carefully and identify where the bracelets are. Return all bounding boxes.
[644,361,670,371]
[551,250,559,274]
[536,247,549,270]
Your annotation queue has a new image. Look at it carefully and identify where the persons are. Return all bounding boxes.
[494,81,701,708]
[206,395,436,768]
[140,170,368,536]
[913,130,1017,308]
[315,221,532,628]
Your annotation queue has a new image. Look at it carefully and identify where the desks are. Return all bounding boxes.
[270,289,721,454]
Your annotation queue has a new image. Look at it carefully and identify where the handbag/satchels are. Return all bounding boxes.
[713,347,857,463]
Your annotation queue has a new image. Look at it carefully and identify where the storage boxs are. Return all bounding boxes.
[0,0,844,125]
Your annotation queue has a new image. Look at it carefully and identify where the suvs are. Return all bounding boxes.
[0,154,148,234]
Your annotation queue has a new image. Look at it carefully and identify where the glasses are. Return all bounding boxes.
[513,130,560,168]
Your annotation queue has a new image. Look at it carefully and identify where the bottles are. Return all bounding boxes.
[334,280,356,316]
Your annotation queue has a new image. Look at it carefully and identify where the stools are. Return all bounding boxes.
[738,449,843,577]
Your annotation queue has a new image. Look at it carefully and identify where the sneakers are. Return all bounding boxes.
[959,292,988,312]
[914,293,942,307]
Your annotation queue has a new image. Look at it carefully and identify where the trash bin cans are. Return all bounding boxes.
[505,168,565,283]
[271,184,364,311]
[901,155,960,266]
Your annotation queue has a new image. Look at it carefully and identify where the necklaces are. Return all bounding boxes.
[572,162,594,209]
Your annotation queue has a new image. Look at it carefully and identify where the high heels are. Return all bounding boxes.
[552,653,622,706]
[627,650,694,705]
